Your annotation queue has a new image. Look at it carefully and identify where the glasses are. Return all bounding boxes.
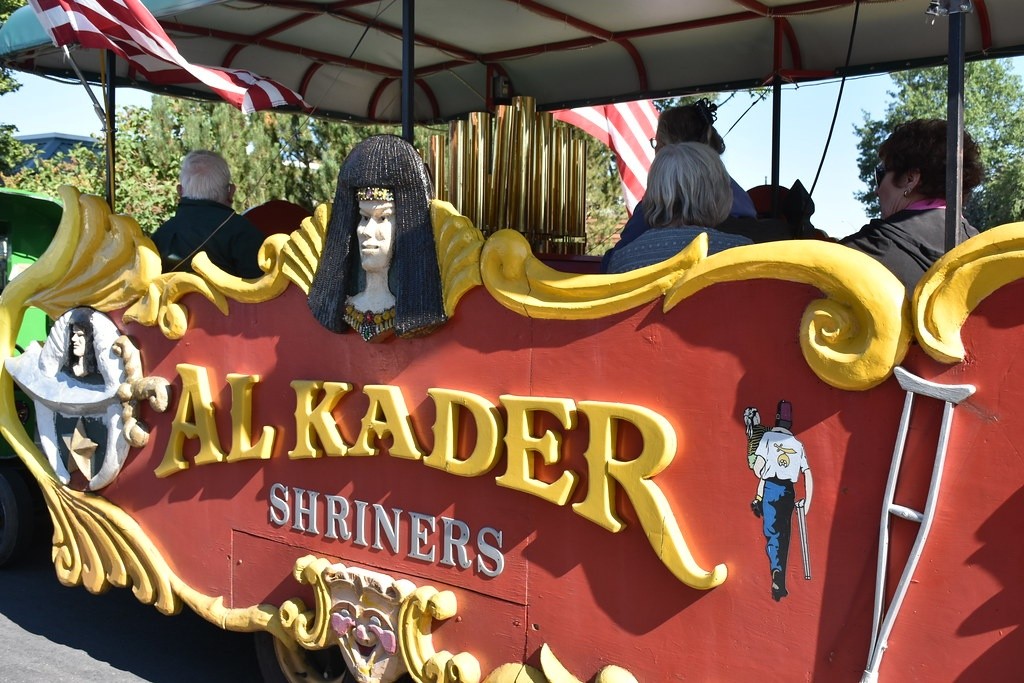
[875,167,899,186]
[650,137,661,149]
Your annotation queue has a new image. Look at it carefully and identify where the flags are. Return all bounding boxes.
[28,0,315,113]
[550,99,660,217]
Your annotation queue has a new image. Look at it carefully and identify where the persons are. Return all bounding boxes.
[152,150,266,279]
[600,105,986,301]
